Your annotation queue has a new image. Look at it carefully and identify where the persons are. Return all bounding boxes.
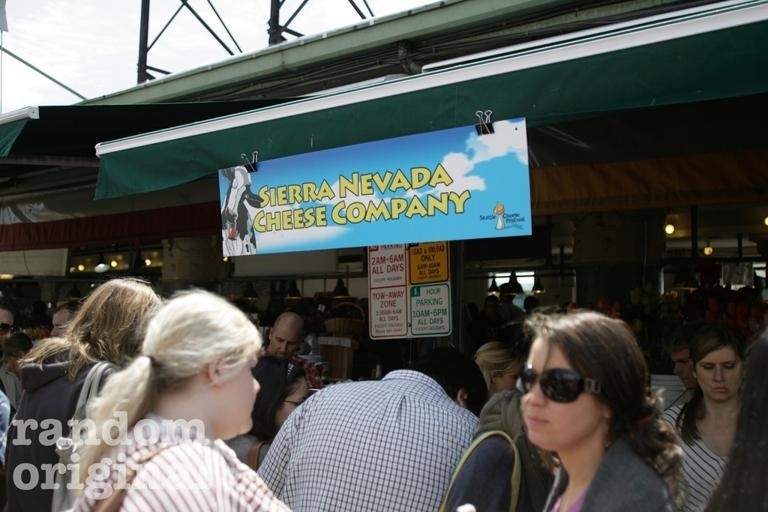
[0,274,768,512]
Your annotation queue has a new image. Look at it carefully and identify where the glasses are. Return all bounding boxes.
[1,323,15,332]
[521,368,601,403]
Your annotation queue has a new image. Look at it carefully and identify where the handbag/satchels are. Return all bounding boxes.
[51,429,87,511]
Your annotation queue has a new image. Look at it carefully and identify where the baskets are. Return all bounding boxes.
[324,302,365,333]
